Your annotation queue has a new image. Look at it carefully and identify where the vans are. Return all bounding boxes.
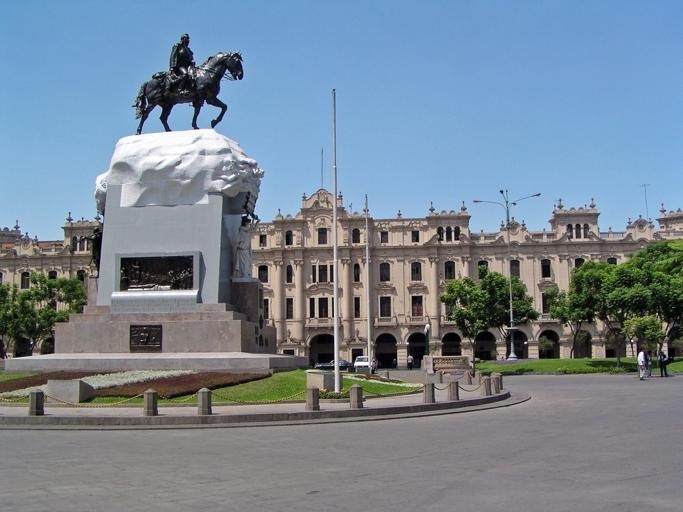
[353,356,376,373]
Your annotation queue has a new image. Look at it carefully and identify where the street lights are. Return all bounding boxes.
[471,188,541,362]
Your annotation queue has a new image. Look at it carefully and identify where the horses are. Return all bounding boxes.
[131,50,244,135]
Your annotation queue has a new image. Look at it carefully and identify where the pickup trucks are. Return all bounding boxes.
[313,359,352,369]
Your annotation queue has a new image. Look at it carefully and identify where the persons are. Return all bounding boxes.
[637,348,646,380]
[169,35,194,94]
[233,216,260,277]
[81,226,103,277]
[658,352,669,377]
[647,350,652,376]
[407,354,413,370]
